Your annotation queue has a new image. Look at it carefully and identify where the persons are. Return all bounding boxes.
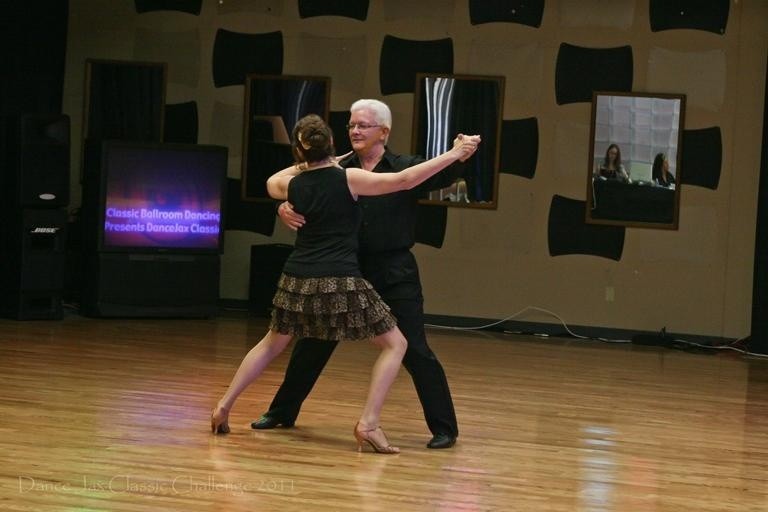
[593,142,633,184]
[250,99,483,449]
[652,152,676,189]
[210,114,483,454]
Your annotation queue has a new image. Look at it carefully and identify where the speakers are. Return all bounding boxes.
[5,110,72,208]
[0,208,66,321]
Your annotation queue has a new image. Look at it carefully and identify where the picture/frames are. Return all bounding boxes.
[411,73,506,210]
[241,73,331,203]
[583,90,687,230]
[79,58,167,184]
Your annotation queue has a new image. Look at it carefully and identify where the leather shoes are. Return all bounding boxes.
[427,431,457,448]
[251,412,296,428]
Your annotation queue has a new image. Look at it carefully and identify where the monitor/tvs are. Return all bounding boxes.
[99,140,228,256]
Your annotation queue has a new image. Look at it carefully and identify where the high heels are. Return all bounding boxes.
[354,423,401,454]
[211,405,231,435]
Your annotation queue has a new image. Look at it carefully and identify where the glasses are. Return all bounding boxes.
[346,124,384,130]
